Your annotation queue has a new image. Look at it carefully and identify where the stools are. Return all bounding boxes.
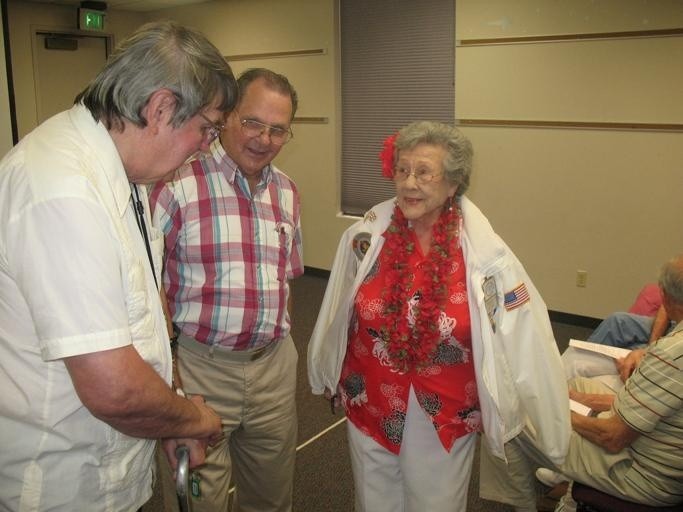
[571,481,683,512]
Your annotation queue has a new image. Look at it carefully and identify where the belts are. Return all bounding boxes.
[176,331,278,362]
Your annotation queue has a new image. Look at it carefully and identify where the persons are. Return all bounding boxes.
[579,299,676,350]
[476,251,683,512]
[150,69,307,512]
[0,19,243,512]
[306,120,572,512]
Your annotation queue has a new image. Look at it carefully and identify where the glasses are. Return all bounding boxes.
[390,167,442,185]
[235,109,294,146]
[199,114,225,145]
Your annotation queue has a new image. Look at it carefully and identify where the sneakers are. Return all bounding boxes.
[535,468,570,488]
